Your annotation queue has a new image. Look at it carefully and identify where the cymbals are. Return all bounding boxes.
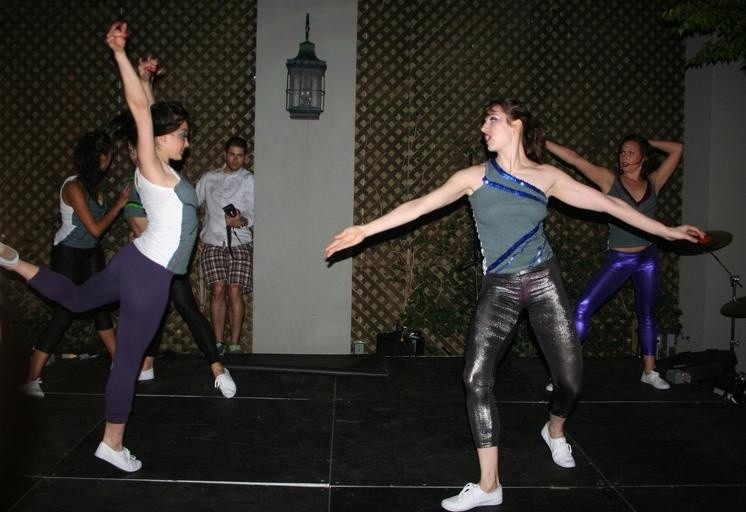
[654,230,733,257]
[719,296,746,318]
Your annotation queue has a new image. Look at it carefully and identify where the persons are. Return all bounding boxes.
[193,138,253,356]
[123,138,237,398]
[0,52,196,473]
[18,129,132,397]
[539,135,682,389]
[326,99,704,512]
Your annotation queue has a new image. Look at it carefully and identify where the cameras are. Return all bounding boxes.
[222,204,237,218]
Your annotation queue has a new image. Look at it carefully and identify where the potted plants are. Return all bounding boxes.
[654,307,685,359]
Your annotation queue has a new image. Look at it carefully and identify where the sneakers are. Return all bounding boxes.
[441,482,503,512]
[93,441,142,472]
[228,344,243,352]
[214,368,237,399]
[541,420,576,468]
[138,369,154,381]
[24,378,45,398]
[546,383,553,392]
[217,342,226,357]
[0,241,19,265]
[640,370,670,390]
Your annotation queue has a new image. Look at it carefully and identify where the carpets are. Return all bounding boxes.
[0,354,746,512]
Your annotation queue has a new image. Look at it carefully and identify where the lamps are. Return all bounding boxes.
[285,13,327,120]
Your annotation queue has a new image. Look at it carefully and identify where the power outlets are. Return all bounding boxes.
[354,340,365,354]
